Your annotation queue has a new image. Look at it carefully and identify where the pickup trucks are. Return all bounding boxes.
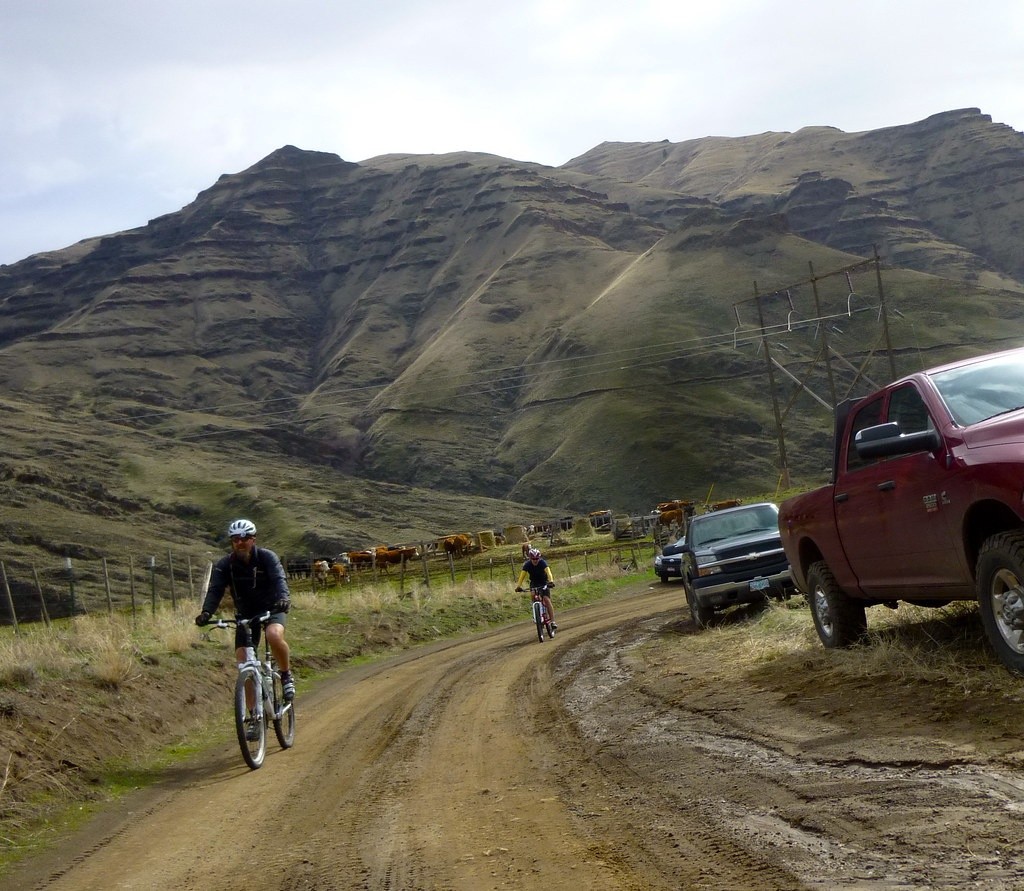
[776,344,1024,676]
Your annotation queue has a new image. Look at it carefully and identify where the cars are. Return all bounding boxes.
[653,534,688,583]
[540,532,551,537]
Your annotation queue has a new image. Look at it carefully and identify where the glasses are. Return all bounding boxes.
[230,535,253,543]
[531,557,539,561]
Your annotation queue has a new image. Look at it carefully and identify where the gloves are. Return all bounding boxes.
[274,599,291,613]
[196,612,212,627]
[515,587,521,592]
[547,582,553,588]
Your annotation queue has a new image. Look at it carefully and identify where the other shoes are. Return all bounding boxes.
[551,622,557,629]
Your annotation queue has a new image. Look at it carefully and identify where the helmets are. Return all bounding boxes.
[528,549,541,558]
[228,520,257,538]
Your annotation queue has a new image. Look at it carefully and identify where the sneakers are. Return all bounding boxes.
[246,708,260,738]
[280,675,295,700]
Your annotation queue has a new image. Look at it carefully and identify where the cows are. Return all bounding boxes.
[337,551,376,572]
[443,538,454,558]
[453,534,470,557]
[657,503,695,531]
[589,509,612,528]
[561,517,573,531]
[315,560,329,585]
[331,564,347,581]
[713,499,743,512]
[287,555,310,579]
[368,545,420,575]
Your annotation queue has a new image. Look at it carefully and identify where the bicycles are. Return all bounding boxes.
[519,586,557,642]
[204,615,295,770]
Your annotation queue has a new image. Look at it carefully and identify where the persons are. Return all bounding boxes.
[195,518,297,742]
[515,548,558,630]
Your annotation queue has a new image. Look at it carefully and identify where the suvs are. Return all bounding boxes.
[661,502,796,629]
